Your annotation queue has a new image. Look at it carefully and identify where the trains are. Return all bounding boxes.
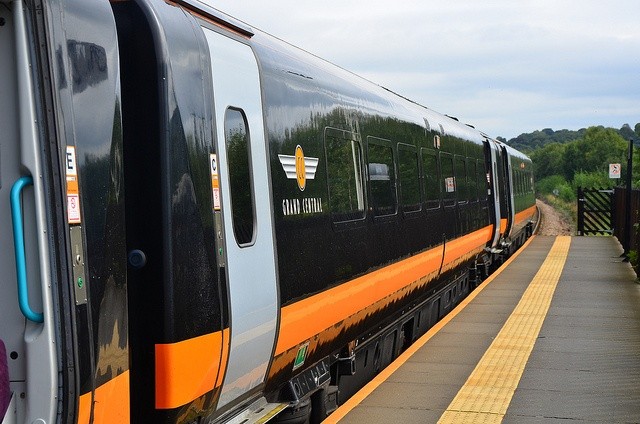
[0,0,538,423]
